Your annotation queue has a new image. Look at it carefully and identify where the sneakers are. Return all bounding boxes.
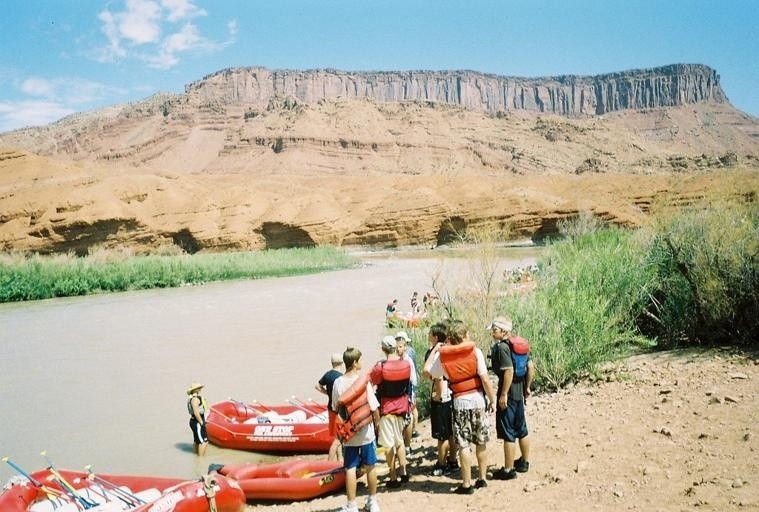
[424,457,528,494]
[335,430,419,512]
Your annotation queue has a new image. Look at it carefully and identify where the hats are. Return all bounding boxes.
[187,382,205,395]
[331,354,343,363]
[396,331,411,342]
[381,336,396,349]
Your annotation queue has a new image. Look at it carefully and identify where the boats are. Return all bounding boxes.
[385,308,446,326]
[0,465,242,512]
[215,455,370,503]
[455,256,553,301]
[200,394,355,453]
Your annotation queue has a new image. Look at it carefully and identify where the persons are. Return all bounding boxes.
[504,264,540,284]
[422,315,535,495]
[386,299,399,313]
[315,332,419,512]
[411,291,439,312]
[187,382,209,456]
